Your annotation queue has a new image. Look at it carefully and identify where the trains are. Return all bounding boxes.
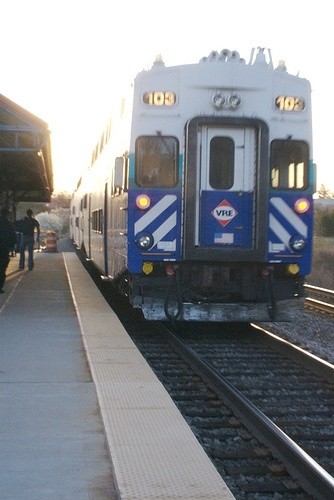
[70,44,315,328]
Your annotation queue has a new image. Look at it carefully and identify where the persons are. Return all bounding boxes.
[0,206,16,292]
[16,208,42,272]
